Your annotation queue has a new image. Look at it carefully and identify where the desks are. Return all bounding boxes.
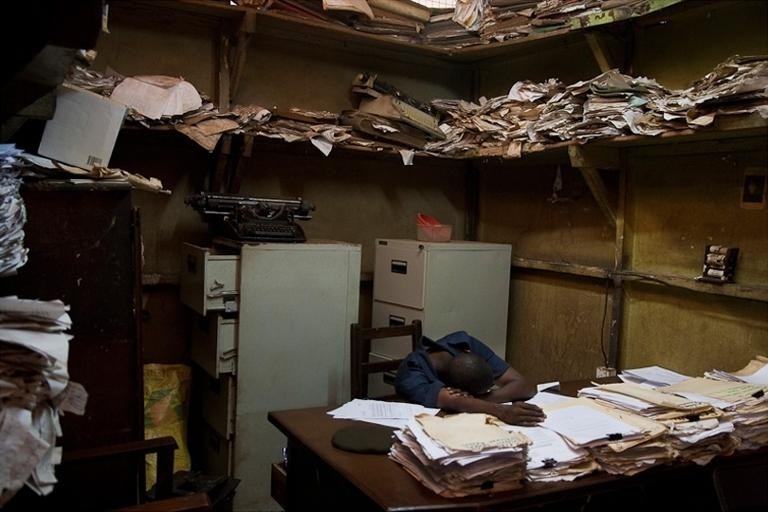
[269,375,622,511]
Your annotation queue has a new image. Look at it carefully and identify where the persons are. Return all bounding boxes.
[394,329,547,428]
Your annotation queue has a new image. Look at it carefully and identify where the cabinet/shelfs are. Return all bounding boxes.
[365,237,513,399]
[1,180,146,512]
[179,240,362,511]
